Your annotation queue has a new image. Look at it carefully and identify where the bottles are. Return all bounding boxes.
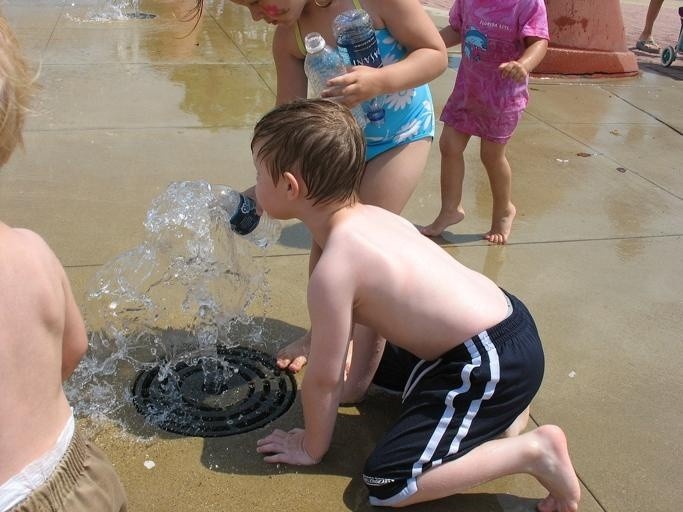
[330,8,388,129]
[302,30,367,136]
[191,181,281,249]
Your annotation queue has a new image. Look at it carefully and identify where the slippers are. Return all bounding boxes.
[636,40,660,54]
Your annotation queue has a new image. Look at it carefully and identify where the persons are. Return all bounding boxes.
[0,16,132,512]
[247,99,583,511]
[638,1,669,53]
[411,0,547,244]
[229,1,449,373]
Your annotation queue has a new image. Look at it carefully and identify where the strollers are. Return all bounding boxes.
[661,7,683,68]
